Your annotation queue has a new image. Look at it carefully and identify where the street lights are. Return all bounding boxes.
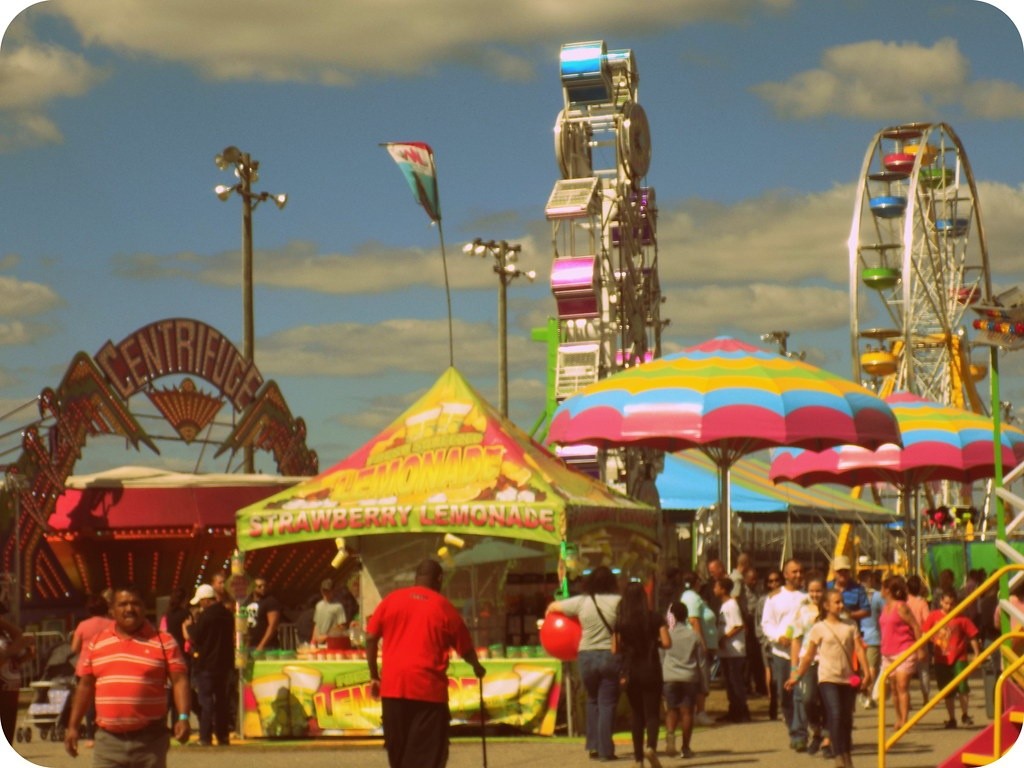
[462,238,539,418]
[210,146,291,474]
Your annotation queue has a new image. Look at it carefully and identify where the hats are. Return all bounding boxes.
[834,555,851,571]
[189,584,214,605]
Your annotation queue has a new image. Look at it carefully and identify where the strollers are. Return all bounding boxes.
[16,643,88,744]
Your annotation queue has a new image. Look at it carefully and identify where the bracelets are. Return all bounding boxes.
[794,674,802,681]
[177,712,188,720]
[791,666,798,671]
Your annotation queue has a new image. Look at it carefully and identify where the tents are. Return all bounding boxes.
[230,365,664,740]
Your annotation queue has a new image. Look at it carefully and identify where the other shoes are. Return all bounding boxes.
[189,740,212,747]
[945,720,958,728]
[791,740,807,750]
[864,700,875,709]
[822,745,835,758]
[644,747,662,768]
[768,703,778,721]
[808,736,823,754]
[843,752,853,768]
[717,704,751,722]
[666,732,676,756]
[600,754,617,762]
[693,712,715,724]
[591,748,599,756]
[636,761,643,767]
[835,755,844,768]
[681,747,693,758]
[219,739,229,747]
[962,714,974,724]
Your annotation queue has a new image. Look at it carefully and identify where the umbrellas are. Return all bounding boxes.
[542,332,1023,588]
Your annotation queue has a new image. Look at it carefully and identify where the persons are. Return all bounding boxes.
[0,552,1024,768]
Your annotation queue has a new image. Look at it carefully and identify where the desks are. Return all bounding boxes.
[244,656,574,737]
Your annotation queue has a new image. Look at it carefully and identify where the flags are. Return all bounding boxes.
[389,139,442,225]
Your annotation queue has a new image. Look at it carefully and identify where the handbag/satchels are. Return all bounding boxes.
[850,672,867,691]
[872,664,891,701]
